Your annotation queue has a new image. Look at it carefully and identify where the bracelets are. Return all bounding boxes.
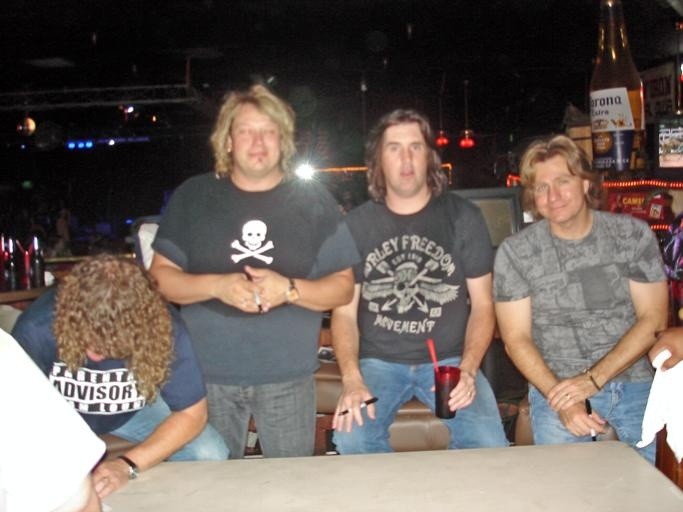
[584,369,603,394]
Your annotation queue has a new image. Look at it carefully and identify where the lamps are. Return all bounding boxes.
[433,72,450,148]
[457,79,473,149]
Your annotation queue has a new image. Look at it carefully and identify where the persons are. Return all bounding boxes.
[48,208,112,258]
[11,254,232,500]
[1,328,109,512]
[649,328,683,371]
[326,109,512,458]
[490,136,670,469]
[148,83,363,461]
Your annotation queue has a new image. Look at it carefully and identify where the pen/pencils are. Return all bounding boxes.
[247,273,262,310]
[338,397,379,416]
[586,399,596,441]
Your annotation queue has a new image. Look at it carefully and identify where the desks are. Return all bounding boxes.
[97,439,683,512]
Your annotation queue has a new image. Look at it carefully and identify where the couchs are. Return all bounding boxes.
[312,327,533,453]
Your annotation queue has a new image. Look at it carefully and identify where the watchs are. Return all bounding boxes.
[283,278,300,305]
[117,455,142,481]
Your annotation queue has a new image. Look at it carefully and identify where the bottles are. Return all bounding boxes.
[3,235,20,284]
[589,0,648,176]
[29,234,43,282]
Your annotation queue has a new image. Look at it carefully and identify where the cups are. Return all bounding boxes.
[434,365,459,418]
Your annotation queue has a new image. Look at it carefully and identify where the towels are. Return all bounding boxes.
[635,348,682,466]
